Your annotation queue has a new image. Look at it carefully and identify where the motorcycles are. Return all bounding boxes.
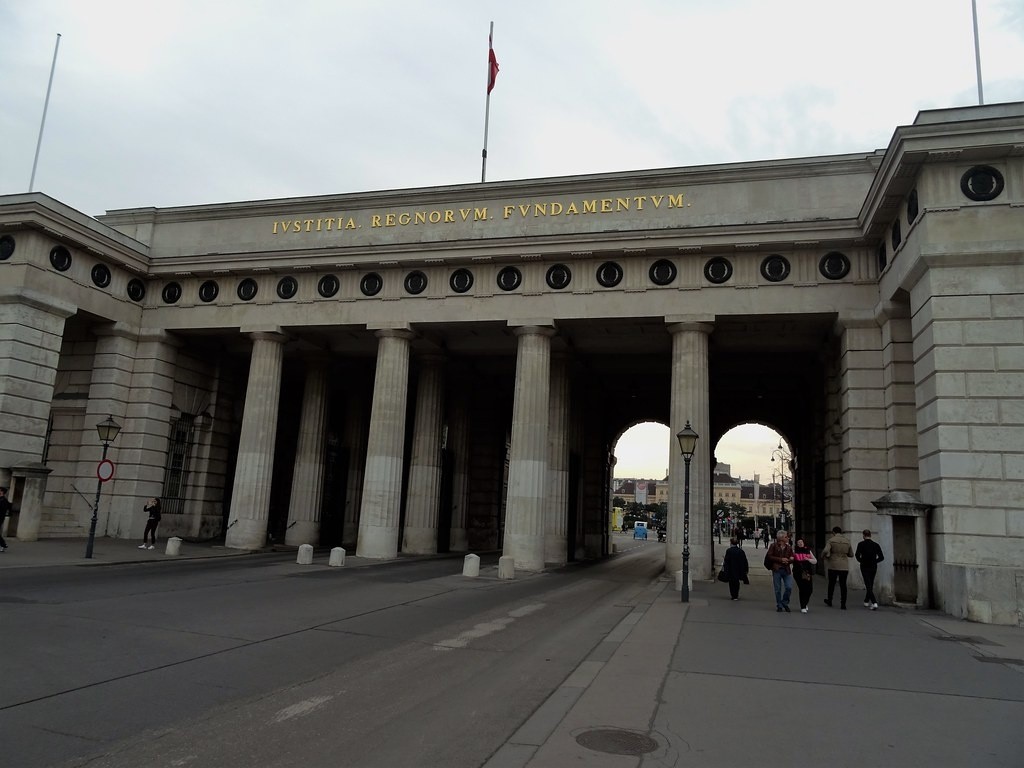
[632,521,649,541]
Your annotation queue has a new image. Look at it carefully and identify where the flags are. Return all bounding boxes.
[488,34,500,96]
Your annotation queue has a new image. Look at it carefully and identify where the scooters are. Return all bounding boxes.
[655,526,667,542]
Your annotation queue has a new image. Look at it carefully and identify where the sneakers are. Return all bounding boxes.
[148,545,155,549]
[138,544,147,548]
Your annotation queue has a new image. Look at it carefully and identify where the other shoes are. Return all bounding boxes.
[870,602,878,610]
[801,605,808,613]
[1,545,8,551]
[824,599,832,607]
[864,602,869,606]
[783,603,791,612]
[777,605,783,612]
[841,605,846,609]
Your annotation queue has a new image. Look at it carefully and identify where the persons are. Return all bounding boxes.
[138,497,161,549]
[765,530,817,613]
[620,523,627,534]
[714,523,778,549]
[855,529,885,610]
[821,527,854,610]
[0,486,9,552]
[722,537,749,600]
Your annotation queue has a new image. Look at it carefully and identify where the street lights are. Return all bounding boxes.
[84,415,123,558]
[675,420,699,603]
[770,449,784,530]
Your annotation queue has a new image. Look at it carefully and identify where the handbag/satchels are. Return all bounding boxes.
[717,565,730,583]
[764,543,776,570]
